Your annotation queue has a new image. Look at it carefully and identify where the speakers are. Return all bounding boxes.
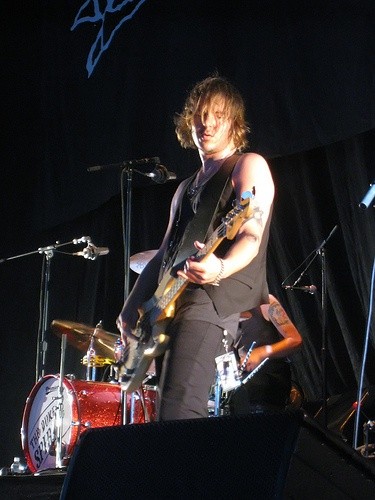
[57,388,375,500]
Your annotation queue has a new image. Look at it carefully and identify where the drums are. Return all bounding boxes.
[21,374,158,473]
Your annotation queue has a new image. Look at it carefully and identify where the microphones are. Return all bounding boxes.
[145,165,178,184]
[285,284,318,294]
[75,242,111,261]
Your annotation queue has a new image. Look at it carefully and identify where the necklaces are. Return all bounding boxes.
[191,176,213,191]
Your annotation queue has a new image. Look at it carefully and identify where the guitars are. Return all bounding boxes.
[116,190,254,393]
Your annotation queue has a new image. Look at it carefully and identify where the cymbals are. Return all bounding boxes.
[50,319,118,361]
[129,249,159,276]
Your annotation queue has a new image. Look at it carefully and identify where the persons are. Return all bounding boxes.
[116,70,277,420]
[228,286,303,416]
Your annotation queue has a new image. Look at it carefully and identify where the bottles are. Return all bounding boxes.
[206,365,221,417]
[9,457,26,475]
[114,338,122,361]
